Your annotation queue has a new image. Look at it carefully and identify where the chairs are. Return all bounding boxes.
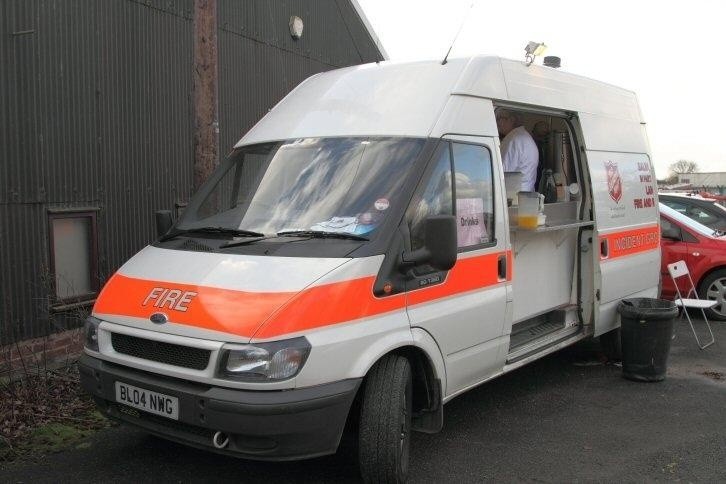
[666,259,719,349]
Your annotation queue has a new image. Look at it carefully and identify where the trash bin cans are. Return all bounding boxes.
[617,298,679,382]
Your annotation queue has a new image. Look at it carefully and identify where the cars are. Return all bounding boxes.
[656,189,725,322]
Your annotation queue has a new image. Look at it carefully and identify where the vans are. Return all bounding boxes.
[76,53,664,483]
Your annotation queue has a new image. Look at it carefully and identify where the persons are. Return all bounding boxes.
[496,106,542,207]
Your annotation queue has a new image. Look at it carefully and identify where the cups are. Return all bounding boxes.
[516,189,545,228]
[537,214,547,225]
